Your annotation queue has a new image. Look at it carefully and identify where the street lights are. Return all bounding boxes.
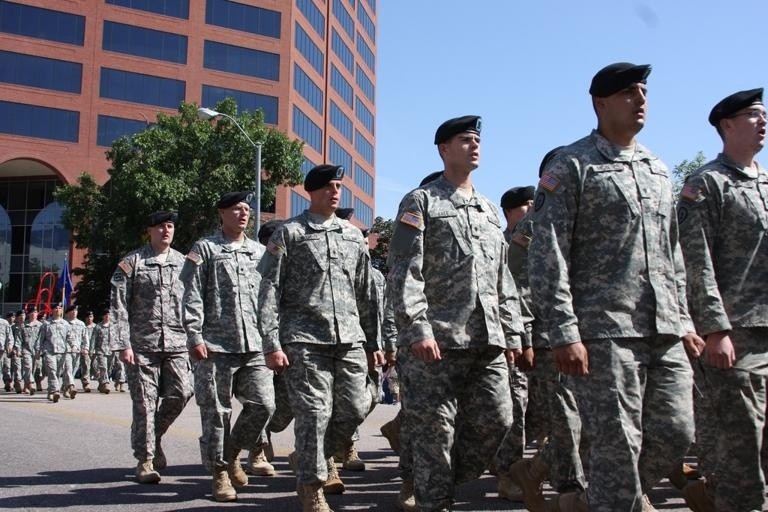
[195,102,265,245]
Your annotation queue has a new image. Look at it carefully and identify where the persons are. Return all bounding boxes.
[108,63,768,512]
[1,302,127,402]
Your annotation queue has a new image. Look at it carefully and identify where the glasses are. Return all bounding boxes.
[730,110,766,119]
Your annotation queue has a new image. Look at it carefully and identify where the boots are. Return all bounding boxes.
[212,436,276,502]
[47,385,77,403]
[84,383,126,394]
[499,471,523,502]
[380,420,400,452]
[152,437,167,470]
[549,491,593,512]
[508,448,551,512]
[680,479,712,512]
[394,477,415,512]
[4,382,42,395]
[135,459,161,484]
[667,462,698,489]
[288,442,365,512]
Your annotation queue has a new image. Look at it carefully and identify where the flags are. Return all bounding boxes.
[57,258,74,311]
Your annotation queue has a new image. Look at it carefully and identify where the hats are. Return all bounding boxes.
[258,220,284,245]
[419,170,444,186]
[708,88,764,125]
[501,185,535,210]
[216,190,254,208]
[102,307,110,315]
[304,165,345,191]
[7,301,62,319]
[65,304,77,314]
[433,115,482,145]
[84,311,94,318]
[588,62,652,98]
[336,207,353,220]
[144,210,176,227]
